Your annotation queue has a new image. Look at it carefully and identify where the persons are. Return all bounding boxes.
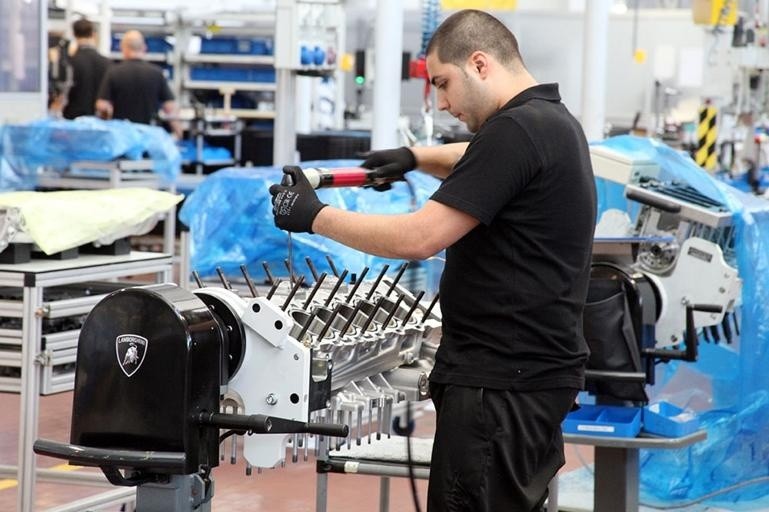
[269,9,598,512]
[63,19,113,119]
[94,29,183,140]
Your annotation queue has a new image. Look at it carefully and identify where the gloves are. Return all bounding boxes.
[268,165,330,234]
[357,145,418,193]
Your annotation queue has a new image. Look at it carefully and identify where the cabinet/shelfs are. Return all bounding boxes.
[95,0,277,122]
[0,159,178,512]
